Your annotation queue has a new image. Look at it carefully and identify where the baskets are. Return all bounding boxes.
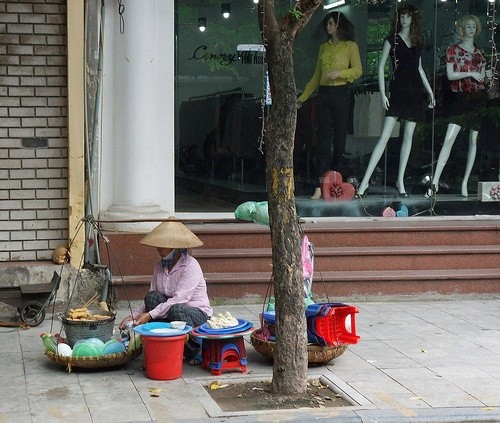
[44,338,142,371]
[249,327,349,367]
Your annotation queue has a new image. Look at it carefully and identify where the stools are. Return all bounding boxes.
[201,335,247,375]
[305,302,362,347]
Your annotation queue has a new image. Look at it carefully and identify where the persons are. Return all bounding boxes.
[355,6,437,198]
[424,14,492,197]
[296,12,363,200]
[119,216,212,365]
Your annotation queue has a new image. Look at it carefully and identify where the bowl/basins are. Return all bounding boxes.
[171,321,187,330]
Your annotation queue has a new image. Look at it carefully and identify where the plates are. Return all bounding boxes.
[133,322,193,336]
[58,338,126,356]
[191,319,255,339]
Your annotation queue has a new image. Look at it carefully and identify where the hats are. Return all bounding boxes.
[139,216,204,249]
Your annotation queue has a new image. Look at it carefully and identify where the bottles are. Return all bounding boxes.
[54,334,65,343]
[40,333,56,353]
[47,333,58,346]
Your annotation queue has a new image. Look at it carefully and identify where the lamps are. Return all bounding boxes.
[221,3,230,18]
[198,17,207,32]
[323,0,350,10]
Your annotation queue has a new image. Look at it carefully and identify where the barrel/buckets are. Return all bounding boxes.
[139,334,189,380]
[59,308,117,350]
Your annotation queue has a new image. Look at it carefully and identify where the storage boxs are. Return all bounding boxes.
[477,181,500,202]
